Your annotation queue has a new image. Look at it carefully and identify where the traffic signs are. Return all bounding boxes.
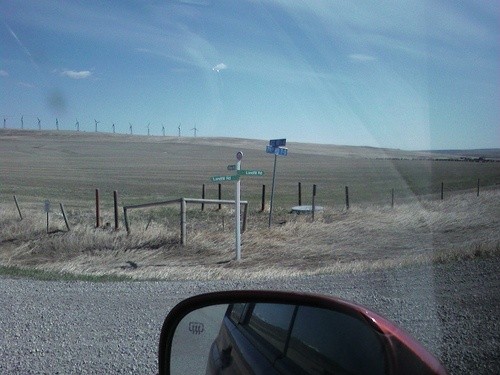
[212,175,240,181]
[269,139,286,147]
[237,169,263,176]
[266,146,287,156]
